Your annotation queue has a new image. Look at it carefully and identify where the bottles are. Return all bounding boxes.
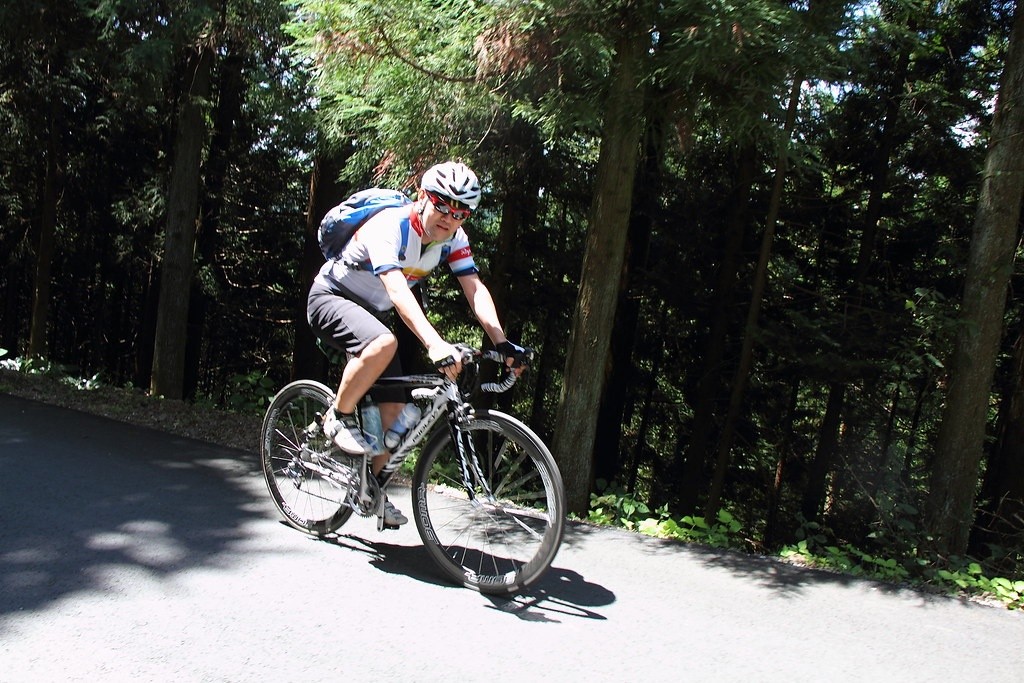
[384,401,421,449]
[360,394,385,458]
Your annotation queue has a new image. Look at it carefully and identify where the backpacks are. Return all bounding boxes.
[318,188,450,271]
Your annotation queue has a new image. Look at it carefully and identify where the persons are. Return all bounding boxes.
[307,162,526,525]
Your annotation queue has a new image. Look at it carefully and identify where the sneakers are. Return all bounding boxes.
[364,484,408,525]
[323,407,373,455]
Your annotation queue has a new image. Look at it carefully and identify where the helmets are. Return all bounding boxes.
[421,162,481,211]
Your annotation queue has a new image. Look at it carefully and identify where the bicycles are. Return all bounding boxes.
[260,344,565,594]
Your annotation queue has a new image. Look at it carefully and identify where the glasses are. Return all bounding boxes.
[424,189,470,220]
